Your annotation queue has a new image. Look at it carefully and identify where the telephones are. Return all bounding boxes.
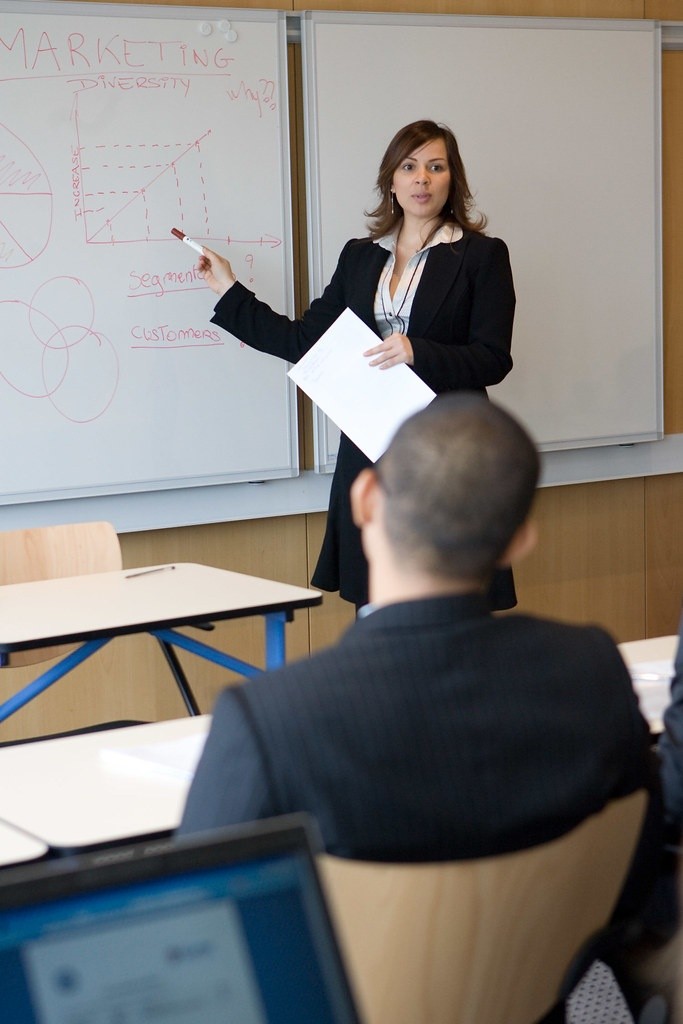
[1,1,301,505]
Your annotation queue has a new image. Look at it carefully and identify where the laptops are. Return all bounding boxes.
[0,809,364,1024]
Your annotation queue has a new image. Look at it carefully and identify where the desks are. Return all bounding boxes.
[0,562,324,727]
[0,635,677,866]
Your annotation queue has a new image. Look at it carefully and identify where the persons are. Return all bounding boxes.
[171,393,683,1024]
[197,121,516,619]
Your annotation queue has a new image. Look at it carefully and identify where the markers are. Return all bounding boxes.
[170,228,204,255]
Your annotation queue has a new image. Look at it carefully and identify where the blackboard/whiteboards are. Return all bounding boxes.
[301,10,665,476]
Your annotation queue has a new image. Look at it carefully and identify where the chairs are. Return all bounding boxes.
[312,791,644,1024]
[0,520,200,748]
